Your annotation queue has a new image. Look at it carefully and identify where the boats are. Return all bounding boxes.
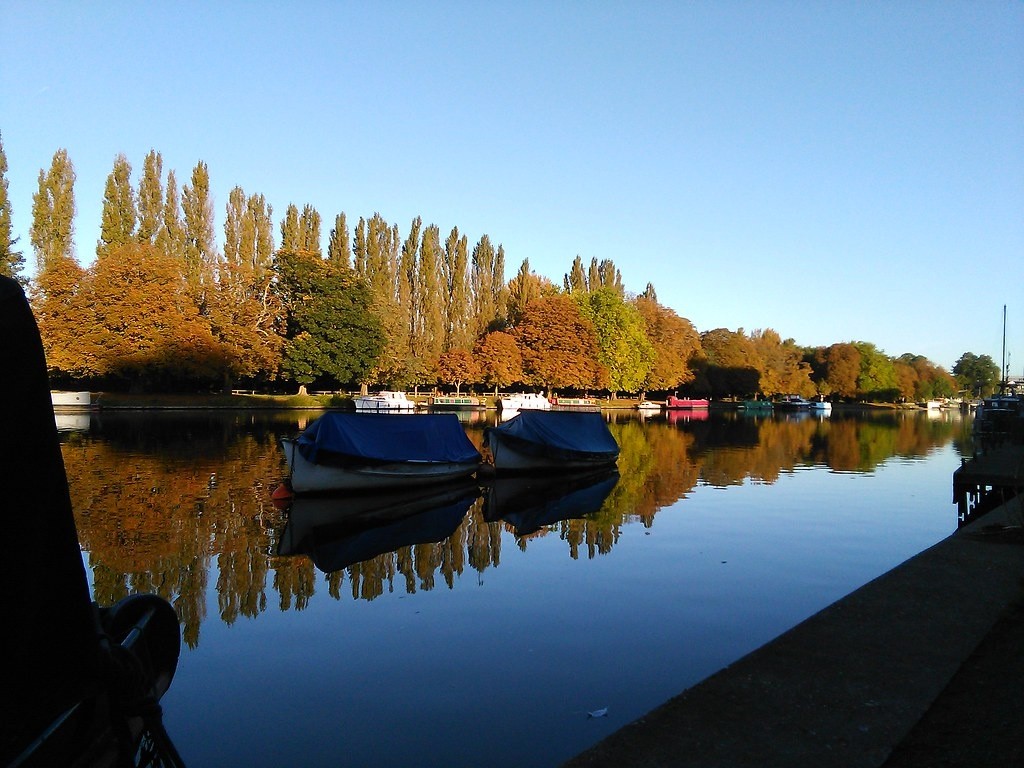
[496,391,551,409]
[486,407,622,468]
[274,477,480,575]
[350,391,414,409]
[638,402,661,409]
[480,470,621,537]
[279,409,484,491]
[665,395,710,408]
[809,401,832,409]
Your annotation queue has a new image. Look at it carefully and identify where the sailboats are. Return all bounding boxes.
[974,303,1021,437]
